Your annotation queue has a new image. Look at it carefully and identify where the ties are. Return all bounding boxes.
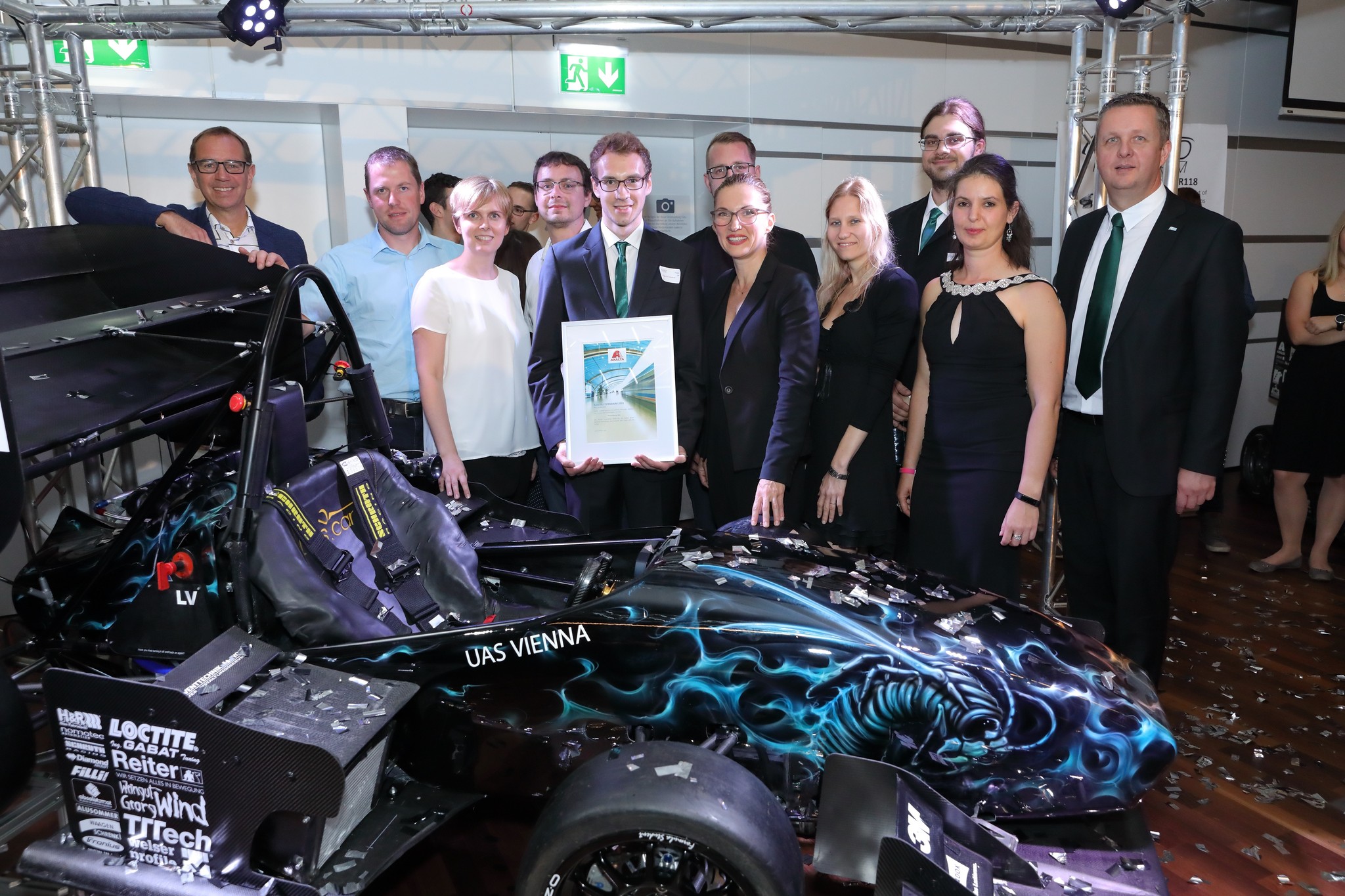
[614,241,632,318]
[919,208,943,255]
[1073,213,1126,401]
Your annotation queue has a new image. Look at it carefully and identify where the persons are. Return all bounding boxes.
[682,131,820,534]
[64,126,327,423]
[1052,92,1256,694]
[410,175,541,522]
[897,153,1066,603]
[815,178,918,562]
[891,96,987,432]
[420,172,462,244]
[494,151,602,513]
[527,131,704,530]
[698,173,819,528]
[1249,211,1345,581]
[240,146,464,459]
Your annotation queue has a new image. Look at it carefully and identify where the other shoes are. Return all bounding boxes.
[1307,555,1335,582]
[1247,553,1303,573]
[1198,535,1231,553]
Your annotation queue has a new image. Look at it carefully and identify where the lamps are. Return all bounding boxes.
[217,0,289,51]
[1095,0,1145,20]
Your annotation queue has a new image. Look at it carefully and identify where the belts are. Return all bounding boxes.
[383,401,422,419]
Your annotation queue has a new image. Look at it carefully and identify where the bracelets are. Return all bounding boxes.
[1015,491,1042,507]
[1335,314,1345,331]
[900,468,917,474]
[828,466,850,480]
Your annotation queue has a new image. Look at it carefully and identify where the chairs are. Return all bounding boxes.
[248,449,488,660]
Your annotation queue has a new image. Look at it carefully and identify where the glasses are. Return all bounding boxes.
[918,135,981,151]
[710,208,770,226]
[534,180,585,194]
[191,159,252,174]
[512,206,536,216]
[594,174,647,192]
[707,162,755,180]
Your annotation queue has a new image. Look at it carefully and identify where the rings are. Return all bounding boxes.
[1013,535,1022,541]
[830,503,836,504]
[897,503,901,507]
